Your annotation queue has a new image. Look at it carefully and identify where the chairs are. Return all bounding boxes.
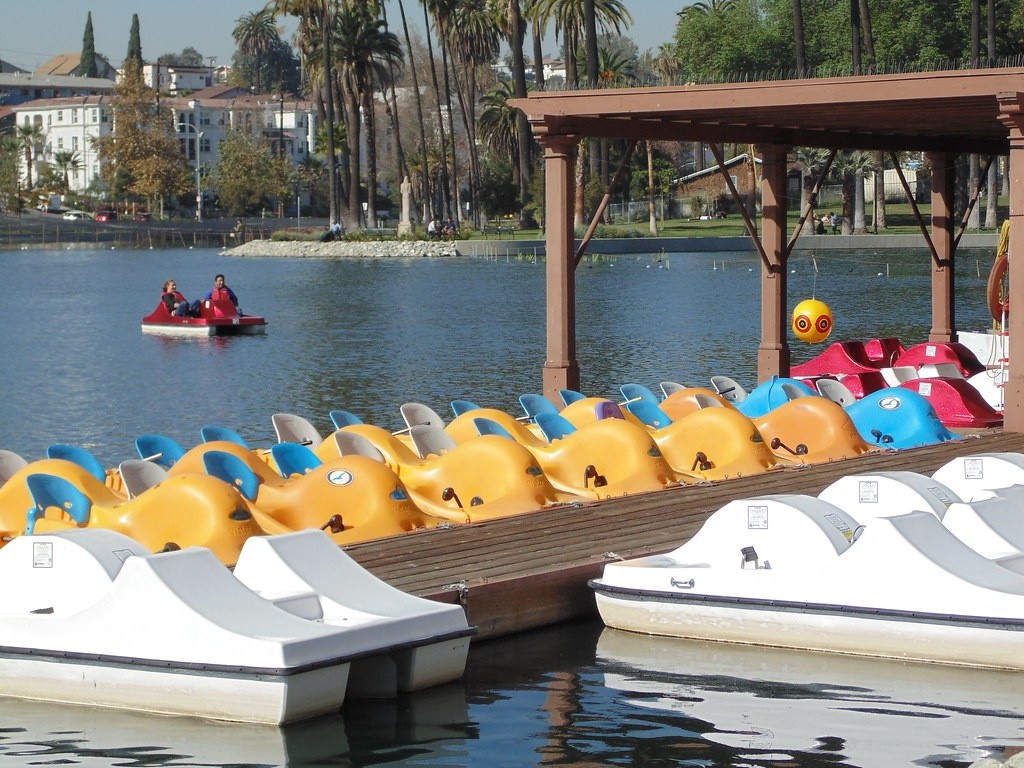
[0,449,28,489]
[270,441,323,479]
[596,402,626,421]
[534,413,577,444]
[473,418,516,440]
[334,431,386,464]
[329,410,364,431]
[134,433,186,471]
[26,473,92,537]
[272,413,323,453]
[558,388,587,410]
[202,450,260,501]
[519,394,560,425]
[620,376,858,429]
[400,403,446,432]
[201,425,251,451]
[450,400,482,418]
[47,444,107,485]
[119,459,171,501]
[409,424,458,460]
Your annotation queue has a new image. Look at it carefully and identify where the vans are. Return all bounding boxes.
[93,211,123,221]
[133,213,159,223]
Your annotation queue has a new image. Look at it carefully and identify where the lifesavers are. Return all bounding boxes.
[987,255,1009,322]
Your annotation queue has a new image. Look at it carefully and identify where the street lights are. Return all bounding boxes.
[175,122,205,221]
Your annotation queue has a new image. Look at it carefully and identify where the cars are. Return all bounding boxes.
[26,190,69,213]
[63,211,91,222]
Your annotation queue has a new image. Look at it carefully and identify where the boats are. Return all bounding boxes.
[141,293,269,340]
[0,336,1024,731]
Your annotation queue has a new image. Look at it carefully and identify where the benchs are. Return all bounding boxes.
[326,227,348,241]
[359,227,397,240]
[484,225,515,239]
[425,228,460,240]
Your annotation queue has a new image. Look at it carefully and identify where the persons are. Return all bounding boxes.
[439,217,455,241]
[821,213,830,223]
[813,211,820,220]
[830,212,841,235]
[427,218,444,242]
[161,280,200,317]
[233,218,246,245]
[716,209,727,219]
[201,274,242,317]
[319,220,341,243]
